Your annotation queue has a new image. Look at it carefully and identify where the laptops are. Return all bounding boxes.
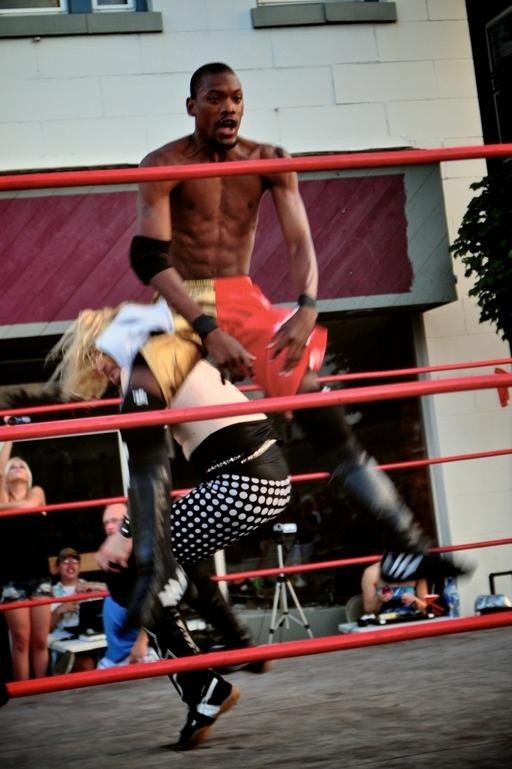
[63,597,106,635]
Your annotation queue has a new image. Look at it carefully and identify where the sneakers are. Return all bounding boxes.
[216,661,273,675]
[377,547,476,585]
[116,552,198,640]
[174,679,240,751]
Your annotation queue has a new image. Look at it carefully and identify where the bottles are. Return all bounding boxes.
[444,576,463,617]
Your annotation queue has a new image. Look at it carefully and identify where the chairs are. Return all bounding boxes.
[345,591,365,623]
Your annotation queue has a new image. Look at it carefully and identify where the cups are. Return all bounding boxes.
[425,593,439,604]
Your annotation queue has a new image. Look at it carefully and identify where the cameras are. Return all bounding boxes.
[8,416,32,425]
[274,522,298,534]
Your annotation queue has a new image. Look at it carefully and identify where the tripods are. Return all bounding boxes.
[268,537,315,646]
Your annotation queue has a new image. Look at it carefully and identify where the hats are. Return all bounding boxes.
[57,547,80,563]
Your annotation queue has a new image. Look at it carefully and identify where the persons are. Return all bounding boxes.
[52,548,111,672]
[47,308,301,747]
[95,505,163,669]
[0,425,50,681]
[363,557,428,616]
[114,64,477,625]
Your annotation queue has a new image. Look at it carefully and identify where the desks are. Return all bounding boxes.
[44,619,210,675]
[337,617,474,636]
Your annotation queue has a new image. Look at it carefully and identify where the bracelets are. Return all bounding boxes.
[191,313,218,338]
[296,293,316,308]
